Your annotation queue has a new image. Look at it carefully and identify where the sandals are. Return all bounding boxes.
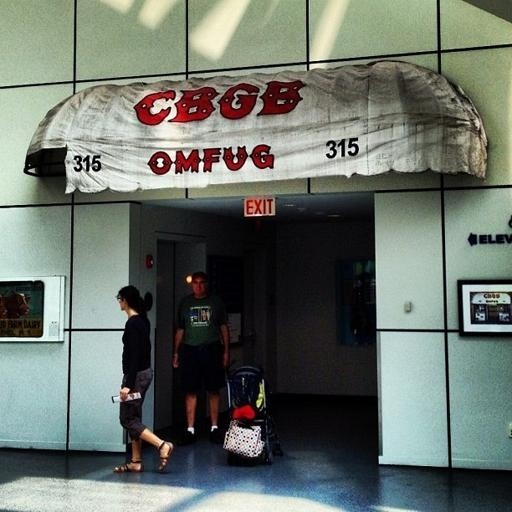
[111,460,145,473]
[157,440,175,471]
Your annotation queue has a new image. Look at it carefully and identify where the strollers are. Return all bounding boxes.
[227,365,283,465]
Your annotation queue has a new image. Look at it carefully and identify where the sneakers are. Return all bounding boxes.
[207,428,225,444]
[182,429,198,445]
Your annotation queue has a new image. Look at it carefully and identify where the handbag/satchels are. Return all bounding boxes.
[223,420,266,458]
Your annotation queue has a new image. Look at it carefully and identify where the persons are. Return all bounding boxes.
[172,271,232,446]
[112,284,176,476]
[1,290,32,319]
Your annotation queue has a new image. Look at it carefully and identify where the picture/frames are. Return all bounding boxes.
[457,280,511,338]
